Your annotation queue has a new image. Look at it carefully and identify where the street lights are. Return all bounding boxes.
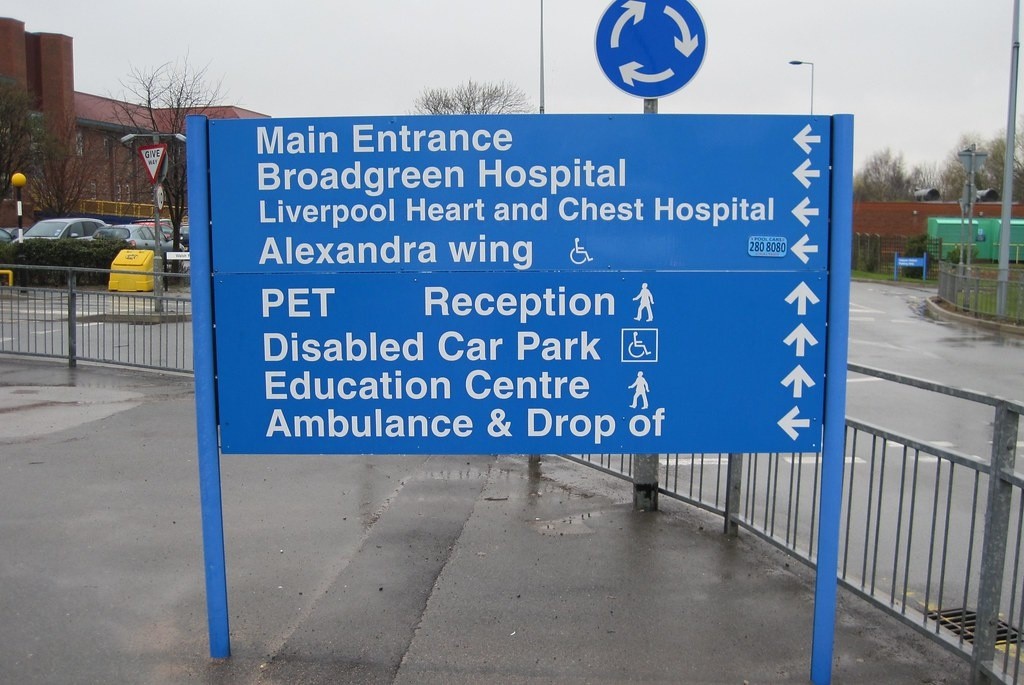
[11,172,26,245]
[790,59,814,115]
[119,132,186,310]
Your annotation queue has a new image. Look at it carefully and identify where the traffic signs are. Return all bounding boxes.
[203,115,832,457]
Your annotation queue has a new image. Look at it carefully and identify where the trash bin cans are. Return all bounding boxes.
[108,249,154,292]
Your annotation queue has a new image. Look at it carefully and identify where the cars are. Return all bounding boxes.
[130,219,189,249]
[0,227,27,245]
[92,224,185,253]
[13,217,107,244]
